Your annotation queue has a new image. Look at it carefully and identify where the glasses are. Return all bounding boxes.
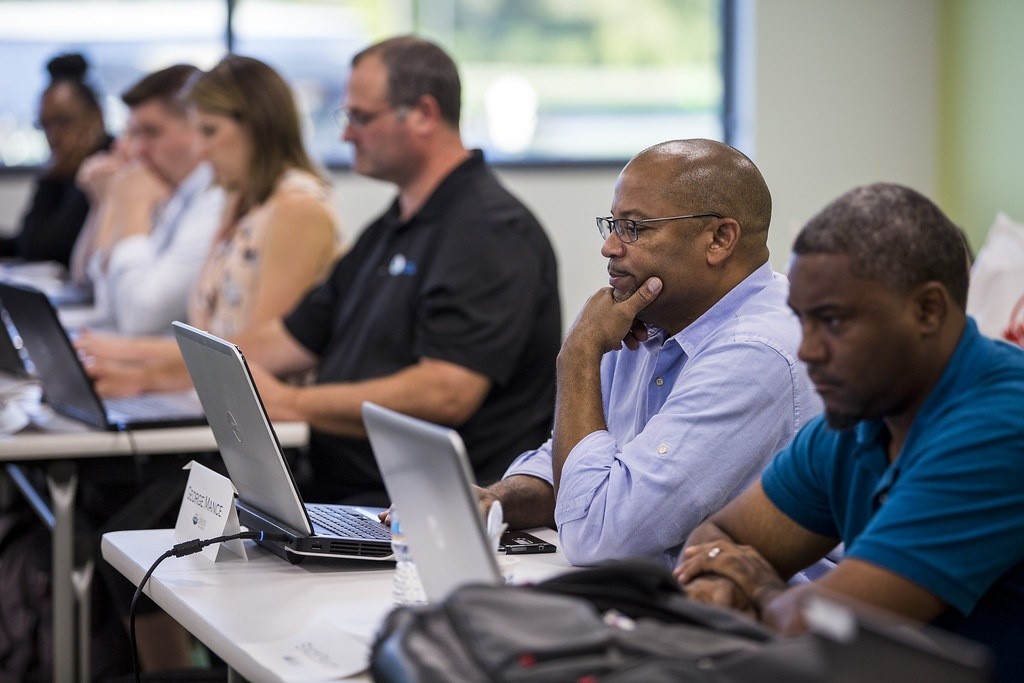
[596,213,728,244]
[33,106,89,130]
[331,98,408,129]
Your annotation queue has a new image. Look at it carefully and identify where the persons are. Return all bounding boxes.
[673,182,1024,683]
[70,65,230,335]
[82,34,562,508]
[0,81,117,262]
[376,140,824,568]
[73,54,344,682]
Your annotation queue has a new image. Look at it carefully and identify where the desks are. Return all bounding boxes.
[0,371,310,683]
[102,522,591,683]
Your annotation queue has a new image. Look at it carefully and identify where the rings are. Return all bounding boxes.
[708,546,721,559]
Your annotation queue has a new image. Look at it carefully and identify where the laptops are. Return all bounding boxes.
[0,280,209,433]
[362,402,507,606]
[0,313,35,379]
[169,321,557,566]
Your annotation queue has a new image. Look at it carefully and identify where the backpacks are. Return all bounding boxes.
[366,556,1024,682]
